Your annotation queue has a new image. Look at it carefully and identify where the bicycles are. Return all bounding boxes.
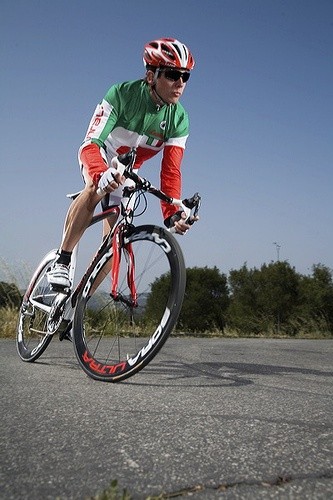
[16,143,203,384]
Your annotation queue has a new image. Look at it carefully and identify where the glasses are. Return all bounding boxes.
[153,68,190,83]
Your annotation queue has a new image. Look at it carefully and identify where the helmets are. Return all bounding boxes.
[143,38,195,70]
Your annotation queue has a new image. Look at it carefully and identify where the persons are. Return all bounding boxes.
[46,38,200,337]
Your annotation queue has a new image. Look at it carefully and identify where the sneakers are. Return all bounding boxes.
[46,258,71,286]
